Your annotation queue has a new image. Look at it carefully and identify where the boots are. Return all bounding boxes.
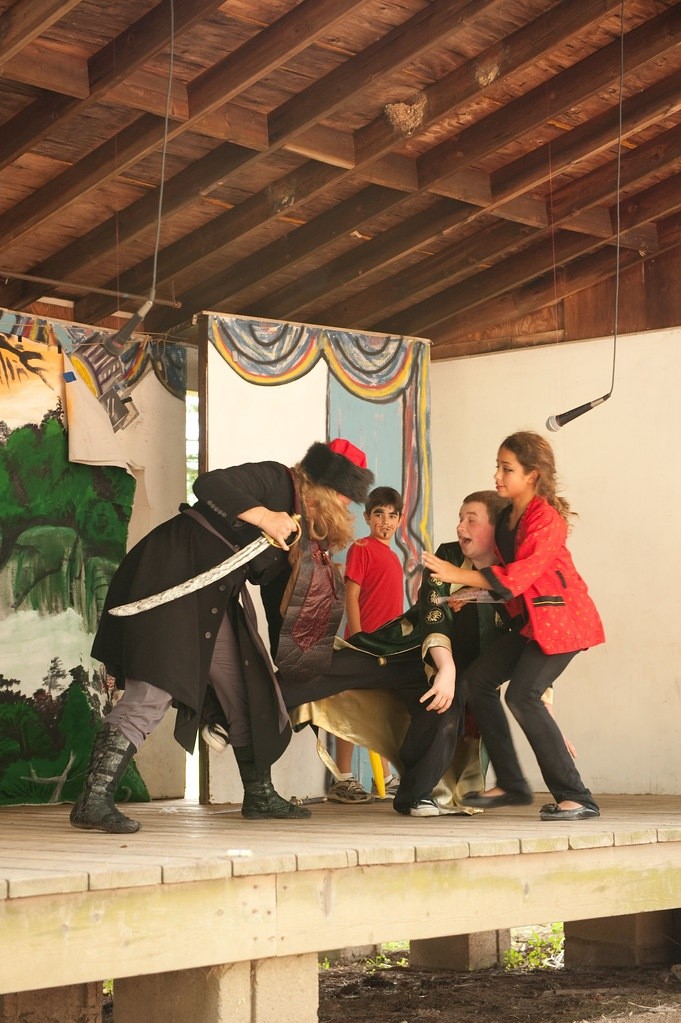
[235,745,314,820]
[70,729,139,833]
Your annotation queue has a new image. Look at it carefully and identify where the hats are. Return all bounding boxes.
[301,440,373,500]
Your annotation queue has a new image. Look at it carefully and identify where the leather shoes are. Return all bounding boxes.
[461,786,532,808]
[541,800,599,821]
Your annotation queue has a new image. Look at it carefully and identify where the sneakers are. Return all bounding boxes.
[395,795,439,815]
[202,719,229,750]
[326,777,375,804]
[370,777,400,799]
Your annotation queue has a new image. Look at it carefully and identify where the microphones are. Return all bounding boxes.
[104,301,153,357]
[546,394,612,432]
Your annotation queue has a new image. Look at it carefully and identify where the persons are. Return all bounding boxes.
[71,432,606,834]
